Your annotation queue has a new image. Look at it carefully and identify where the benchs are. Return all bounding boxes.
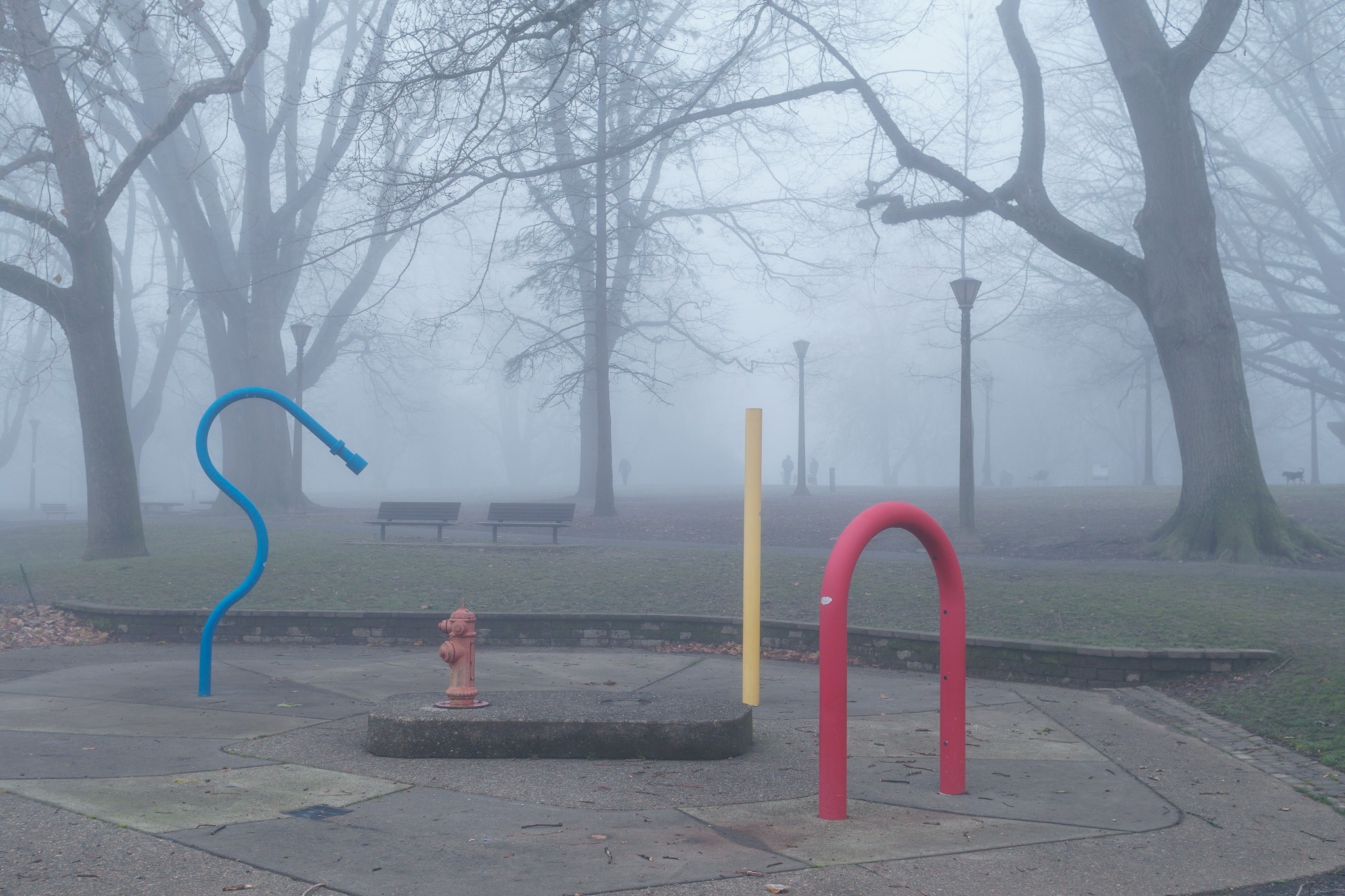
[477,502,575,545]
[364,502,465,542]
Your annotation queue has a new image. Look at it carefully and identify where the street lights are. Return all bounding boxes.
[1307,366,1321,486]
[287,318,312,513]
[1139,344,1156,486]
[25,419,42,513]
[950,274,982,541]
[790,339,812,497]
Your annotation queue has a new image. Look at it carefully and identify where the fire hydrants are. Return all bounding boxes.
[432,594,491,708]
